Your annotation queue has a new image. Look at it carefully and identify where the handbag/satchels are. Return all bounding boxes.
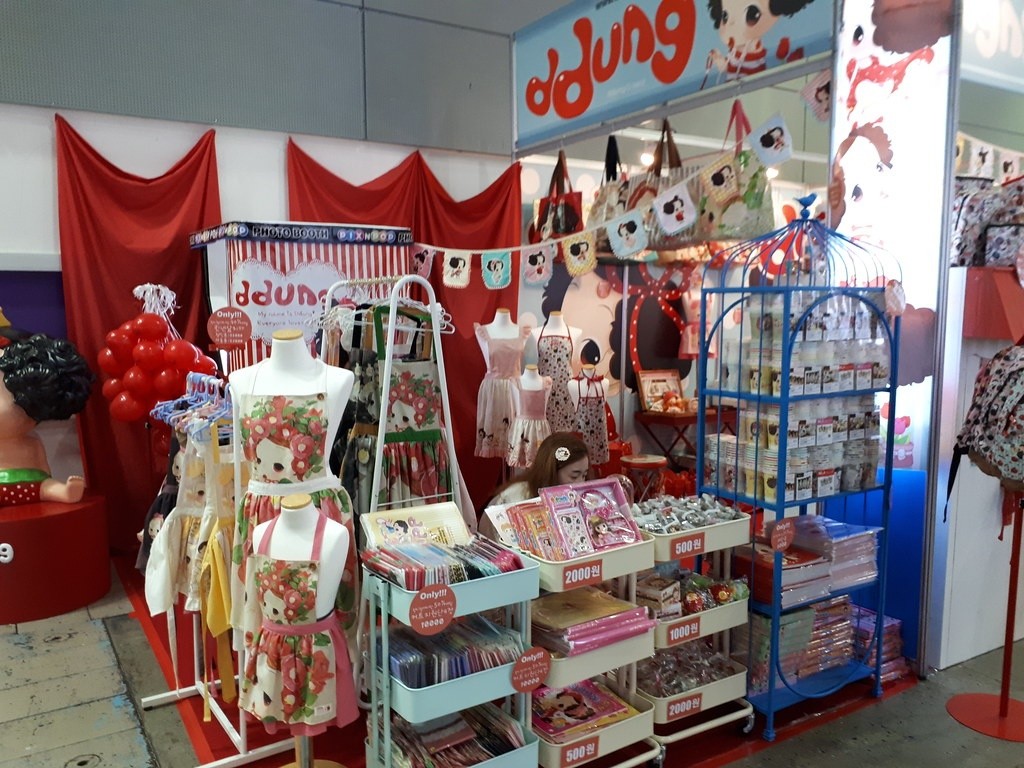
[534,150,584,262]
[949,177,1024,267]
[957,345,1024,481]
[626,118,707,251]
[697,100,775,241]
[583,135,629,252]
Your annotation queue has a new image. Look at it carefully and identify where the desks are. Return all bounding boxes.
[635,406,736,479]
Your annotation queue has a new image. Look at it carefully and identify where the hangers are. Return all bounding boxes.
[149,370,233,441]
[304,274,456,335]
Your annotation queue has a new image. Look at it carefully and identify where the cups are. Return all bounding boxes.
[702,434,880,504]
[718,307,891,397]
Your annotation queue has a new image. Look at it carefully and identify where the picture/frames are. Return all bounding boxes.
[635,369,684,410]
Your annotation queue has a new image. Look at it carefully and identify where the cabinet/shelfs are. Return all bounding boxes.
[360,194,904,768]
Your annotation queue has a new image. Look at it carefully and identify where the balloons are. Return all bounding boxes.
[98,311,217,420]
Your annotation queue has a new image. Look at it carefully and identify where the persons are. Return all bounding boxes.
[229,307,611,735]
[480,432,590,541]
[0,333,95,505]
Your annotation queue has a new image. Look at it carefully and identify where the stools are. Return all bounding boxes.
[620,453,667,503]
